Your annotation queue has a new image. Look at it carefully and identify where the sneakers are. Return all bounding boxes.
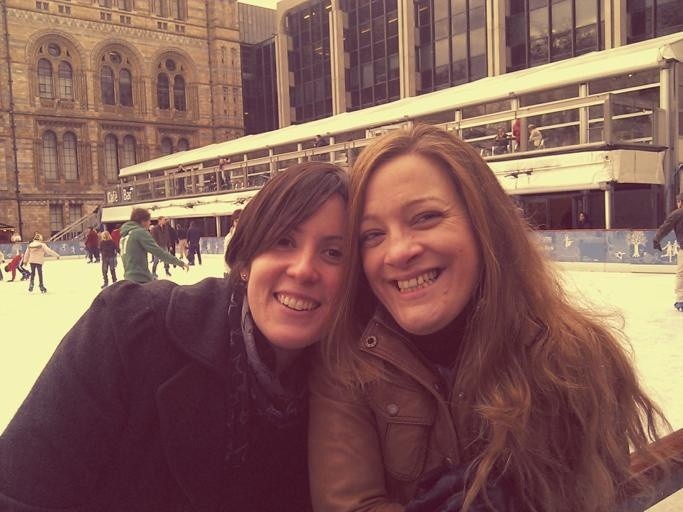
[29,287,46,292]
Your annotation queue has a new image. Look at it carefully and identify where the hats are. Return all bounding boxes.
[33,232,42,240]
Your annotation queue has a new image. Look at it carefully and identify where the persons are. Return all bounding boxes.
[5,255,30,283]
[0,160,355,512]
[203,156,233,192]
[493,116,543,155]
[174,164,187,195]
[305,121,677,512]
[123,177,130,194]
[579,212,591,229]
[81,208,201,289]
[23,231,60,292]
[0,250,5,281]
[313,135,328,161]
[653,193,683,312]
[223,209,241,278]
